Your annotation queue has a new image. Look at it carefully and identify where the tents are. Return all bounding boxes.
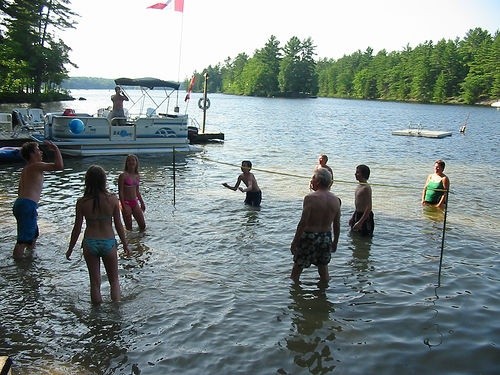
[114,77,179,119]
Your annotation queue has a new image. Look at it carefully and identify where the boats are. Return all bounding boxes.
[29,112,204,158]
[151,73,225,146]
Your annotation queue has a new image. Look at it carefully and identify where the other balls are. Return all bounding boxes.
[68,118,85,135]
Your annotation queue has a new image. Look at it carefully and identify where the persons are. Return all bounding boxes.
[119,155,147,230]
[111,86,129,125]
[291,155,374,280]
[66,165,130,306]
[221,160,261,207]
[13,140,63,259]
[420,161,451,210]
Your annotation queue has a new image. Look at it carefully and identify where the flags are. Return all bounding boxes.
[147,0,184,11]
[185,76,194,102]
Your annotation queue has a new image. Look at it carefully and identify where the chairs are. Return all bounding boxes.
[11,109,46,136]
[0,113,12,123]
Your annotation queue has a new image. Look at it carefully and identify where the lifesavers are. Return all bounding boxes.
[198,96,211,110]
